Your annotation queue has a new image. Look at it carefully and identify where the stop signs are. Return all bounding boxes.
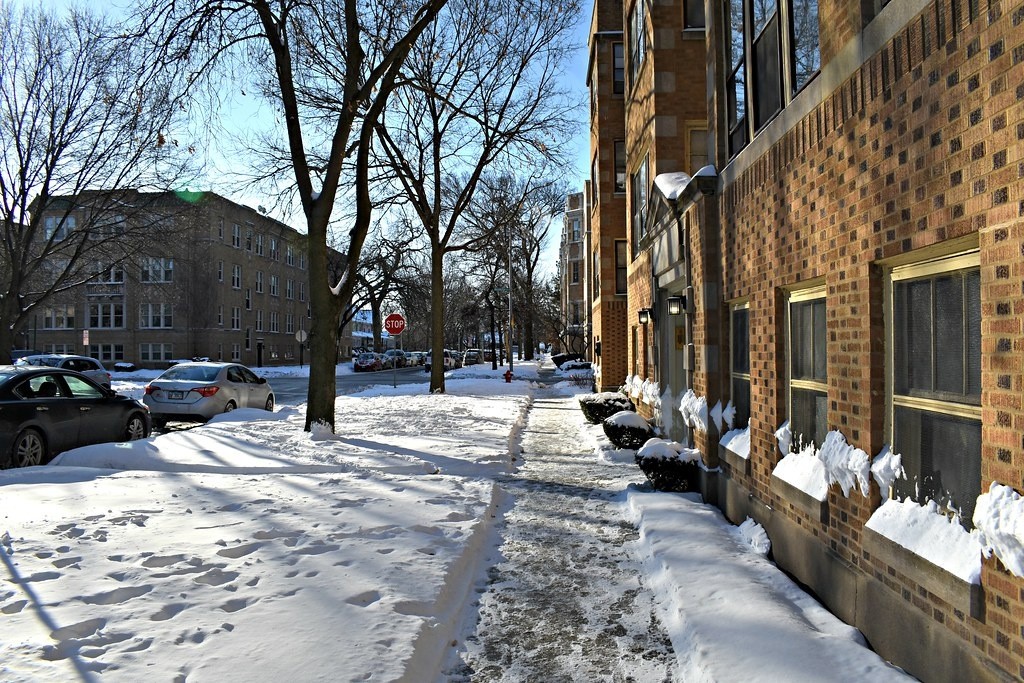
[384,314,406,335]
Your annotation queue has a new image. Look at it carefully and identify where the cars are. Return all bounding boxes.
[353,353,382,371]
[14,354,111,391]
[144,361,276,432]
[383,349,406,369]
[406,348,506,373]
[0,365,152,468]
[377,354,392,370]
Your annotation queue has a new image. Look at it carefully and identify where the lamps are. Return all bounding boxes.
[638,307,655,325]
[665,295,687,317]
